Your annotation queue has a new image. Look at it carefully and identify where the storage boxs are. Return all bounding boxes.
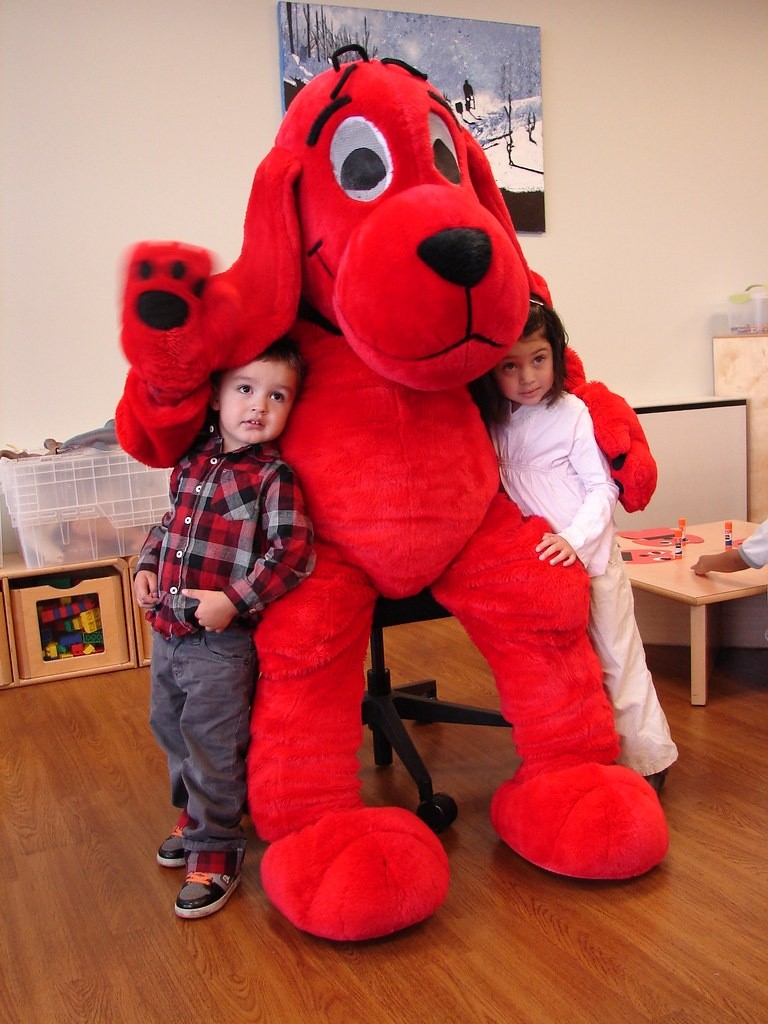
[10,569,130,679]
[1,445,174,569]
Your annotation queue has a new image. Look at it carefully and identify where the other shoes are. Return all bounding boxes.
[645,768,669,794]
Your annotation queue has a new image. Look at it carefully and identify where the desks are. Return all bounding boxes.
[614,519,768,708]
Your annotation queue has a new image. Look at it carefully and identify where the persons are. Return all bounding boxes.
[133,337,317,919]
[465,292,676,797]
[691,520,768,575]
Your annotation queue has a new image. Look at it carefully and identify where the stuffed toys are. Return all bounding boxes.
[116,44,668,941]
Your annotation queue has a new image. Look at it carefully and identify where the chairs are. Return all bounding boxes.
[359,586,512,832]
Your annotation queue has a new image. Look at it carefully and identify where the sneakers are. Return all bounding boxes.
[174,872,242,919]
[156,807,191,867]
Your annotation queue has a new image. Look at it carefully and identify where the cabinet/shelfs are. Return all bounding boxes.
[0,547,154,688]
